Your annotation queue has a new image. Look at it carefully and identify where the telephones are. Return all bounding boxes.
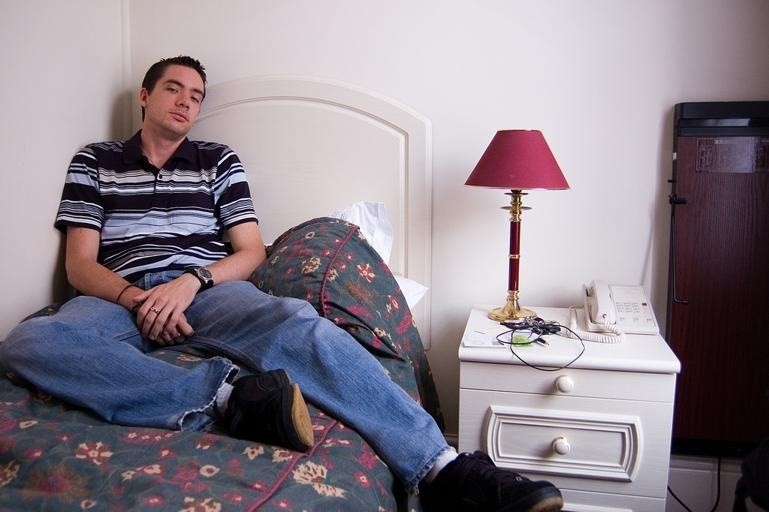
[581,279,660,337]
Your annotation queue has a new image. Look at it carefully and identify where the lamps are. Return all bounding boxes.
[464,129,571,323]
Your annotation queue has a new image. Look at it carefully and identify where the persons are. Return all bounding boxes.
[3,54,564,508]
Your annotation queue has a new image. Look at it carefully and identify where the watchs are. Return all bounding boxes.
[183,265,214,292]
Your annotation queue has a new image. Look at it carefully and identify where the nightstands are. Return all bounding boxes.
[457,307,682,511]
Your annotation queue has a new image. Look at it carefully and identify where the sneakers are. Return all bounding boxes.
[225,369,314,453]
[418,450,563,511]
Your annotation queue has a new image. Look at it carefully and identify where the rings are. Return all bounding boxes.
[149,306,160,314]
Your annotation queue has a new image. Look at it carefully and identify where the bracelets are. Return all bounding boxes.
[115,284,136,304]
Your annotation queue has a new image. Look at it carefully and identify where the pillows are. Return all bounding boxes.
[393,275,429,311]
[328,200,393,268]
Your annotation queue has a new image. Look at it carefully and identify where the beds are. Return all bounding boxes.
[1,74,447,512]
